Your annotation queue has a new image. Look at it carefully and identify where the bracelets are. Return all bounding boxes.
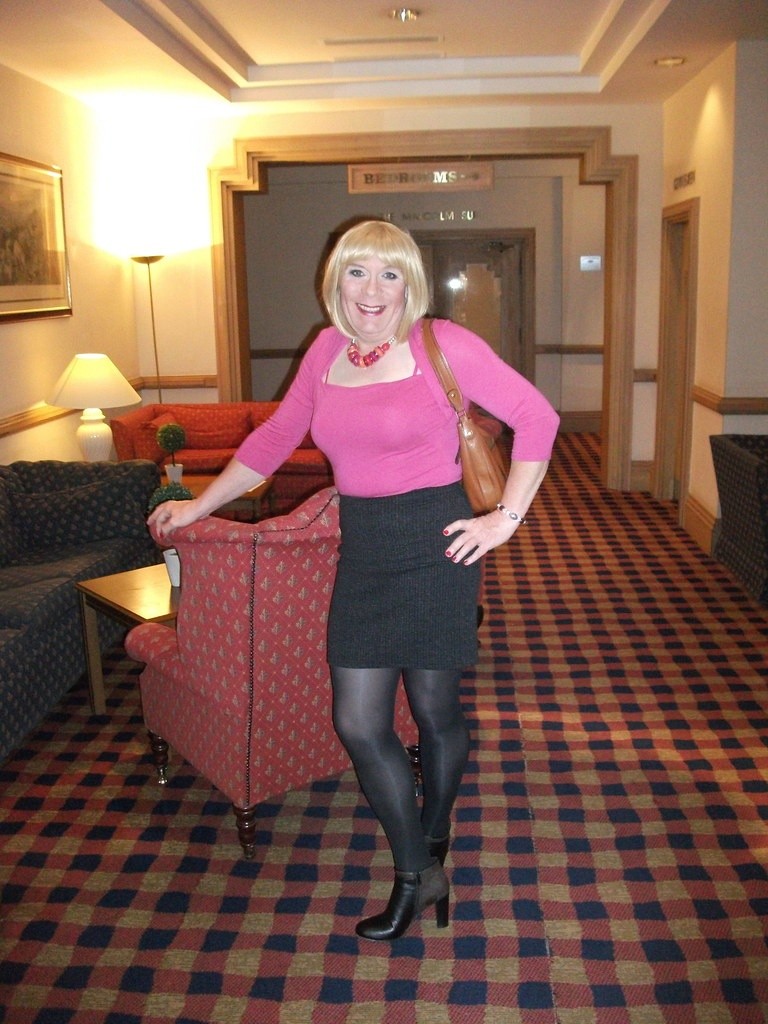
[496,502,528,527]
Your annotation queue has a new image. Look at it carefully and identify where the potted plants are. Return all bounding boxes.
[157,423,186,483]
[150,486,194,587]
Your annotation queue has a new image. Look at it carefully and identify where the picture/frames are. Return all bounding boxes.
[0,151,73,326]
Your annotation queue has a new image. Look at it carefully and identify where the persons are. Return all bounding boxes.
[147,221,560,940]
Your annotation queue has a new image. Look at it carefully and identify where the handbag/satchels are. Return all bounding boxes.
[421,316,509,515]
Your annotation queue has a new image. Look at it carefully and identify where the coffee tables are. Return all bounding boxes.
[162,474,279,522]
[76,562,181,763]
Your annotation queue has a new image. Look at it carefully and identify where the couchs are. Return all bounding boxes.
[110,402,335,521]
[710,434,768,608]
[126,486,419,858]
[0,459,161,760]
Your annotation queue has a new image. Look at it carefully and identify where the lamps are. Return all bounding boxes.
[45,353,142,462]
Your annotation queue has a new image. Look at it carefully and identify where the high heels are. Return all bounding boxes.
[356,857,451,940]
[423,838,456,868]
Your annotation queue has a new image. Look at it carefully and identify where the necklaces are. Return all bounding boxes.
[347,335,396,368]
